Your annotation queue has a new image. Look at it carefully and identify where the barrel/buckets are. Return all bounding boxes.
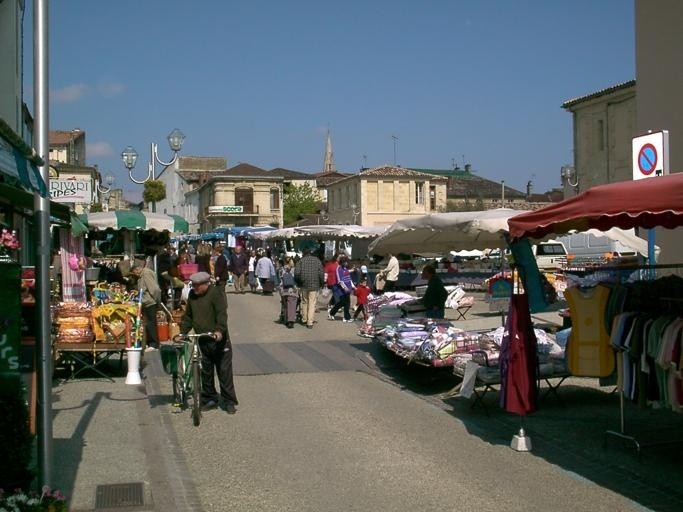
[157,322,169,341]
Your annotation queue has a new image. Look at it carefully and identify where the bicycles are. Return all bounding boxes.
[168,331,216,426]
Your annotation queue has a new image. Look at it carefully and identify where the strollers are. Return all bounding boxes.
[279,293,302,323]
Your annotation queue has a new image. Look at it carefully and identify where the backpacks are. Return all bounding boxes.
[282,272,296,289]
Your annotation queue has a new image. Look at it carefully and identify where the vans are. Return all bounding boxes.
[531,241,568,270]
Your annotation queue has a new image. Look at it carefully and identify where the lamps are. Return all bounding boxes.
[350,204,360,215]
[320,210,330,221]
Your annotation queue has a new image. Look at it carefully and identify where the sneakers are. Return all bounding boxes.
[307,325,313,329]
[342,317,355,323]
[287,321,294,329]
[235,290,258,295]
[201,401,218,411]
[302,322,307,326]
[328,308,334,320]
[219,401,236,414]
[144,346,159,353]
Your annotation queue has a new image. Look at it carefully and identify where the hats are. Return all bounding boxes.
[189,271,211,284]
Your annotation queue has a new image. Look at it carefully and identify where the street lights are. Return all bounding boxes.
[391,135,401,165]
[122,127,186,271]
[92,172,114,205]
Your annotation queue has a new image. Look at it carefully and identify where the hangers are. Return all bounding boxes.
[562,262,658,288]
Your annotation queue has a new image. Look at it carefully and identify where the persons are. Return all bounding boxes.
[419,264,447,320]
[172,272,239,414]
[129,261,162,353]
[165,238,400,330]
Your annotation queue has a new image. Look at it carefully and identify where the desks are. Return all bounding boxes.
[54,342,126,383]
